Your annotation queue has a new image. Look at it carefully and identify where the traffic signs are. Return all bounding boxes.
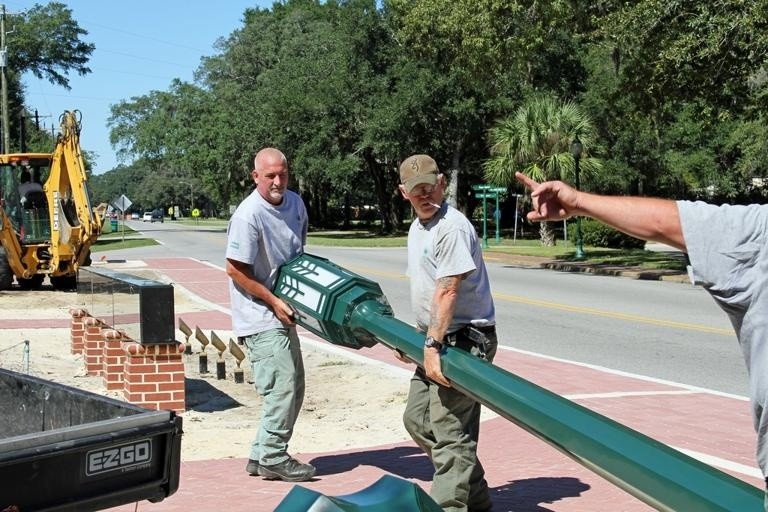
[471,181,506,202]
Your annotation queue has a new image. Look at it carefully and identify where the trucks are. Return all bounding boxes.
[2,367,181,512]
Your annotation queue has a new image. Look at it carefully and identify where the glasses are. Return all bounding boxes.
[404,184,439,197]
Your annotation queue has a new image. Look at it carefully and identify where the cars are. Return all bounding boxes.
[131,212,140,221]
[143,211,153,222]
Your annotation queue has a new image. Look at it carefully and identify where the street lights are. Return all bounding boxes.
[270,253,768,510]
[179,179,194,219]
[567,129,589,257]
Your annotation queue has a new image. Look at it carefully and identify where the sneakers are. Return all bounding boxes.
[245,457,259,475]
[257,457,317,482]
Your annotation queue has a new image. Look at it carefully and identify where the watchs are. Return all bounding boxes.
[425,335,443,352]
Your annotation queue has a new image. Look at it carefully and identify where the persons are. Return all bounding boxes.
[223,147,318,483]
[513,169,767,511]
[16,169,43,200]
[390,154,498,511]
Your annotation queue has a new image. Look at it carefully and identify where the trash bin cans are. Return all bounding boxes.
[110,214,118,232]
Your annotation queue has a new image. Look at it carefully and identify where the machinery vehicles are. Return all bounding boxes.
[1,105,109,291]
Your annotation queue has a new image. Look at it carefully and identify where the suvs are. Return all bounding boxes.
[151,208,165,223]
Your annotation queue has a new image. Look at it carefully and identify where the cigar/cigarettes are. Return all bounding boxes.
[429,201,442,209]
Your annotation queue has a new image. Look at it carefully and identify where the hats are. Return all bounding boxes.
[397,153,441,195]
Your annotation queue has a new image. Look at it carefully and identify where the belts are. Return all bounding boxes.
[444,321,497,345]
